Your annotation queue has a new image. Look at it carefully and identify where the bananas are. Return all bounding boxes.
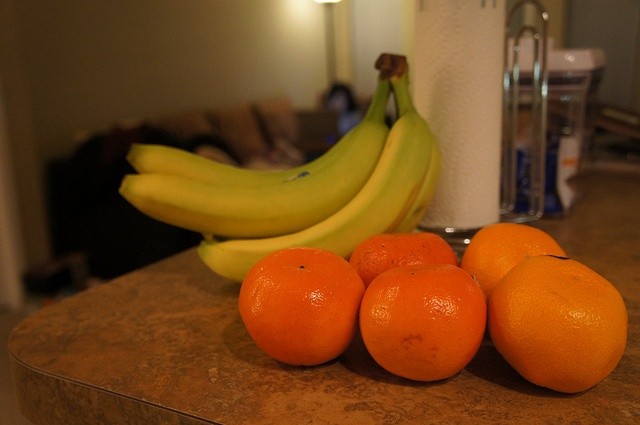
[118,52,438,283]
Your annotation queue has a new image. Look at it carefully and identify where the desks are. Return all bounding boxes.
[7,239,640,425]
